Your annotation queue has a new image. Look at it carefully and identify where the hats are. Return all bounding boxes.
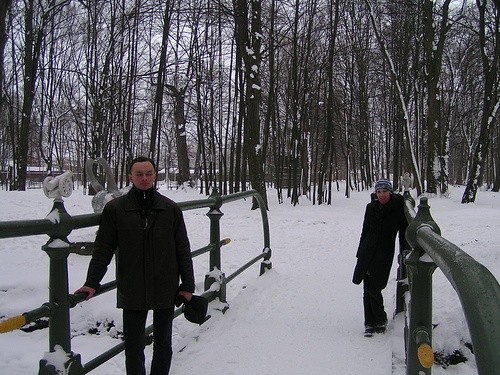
[374,180,393,193]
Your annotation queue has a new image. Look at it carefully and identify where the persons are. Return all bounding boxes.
[352,179,411,337]
[76,156,195,375]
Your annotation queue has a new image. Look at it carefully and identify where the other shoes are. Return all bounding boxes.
[363,325,386,337]
